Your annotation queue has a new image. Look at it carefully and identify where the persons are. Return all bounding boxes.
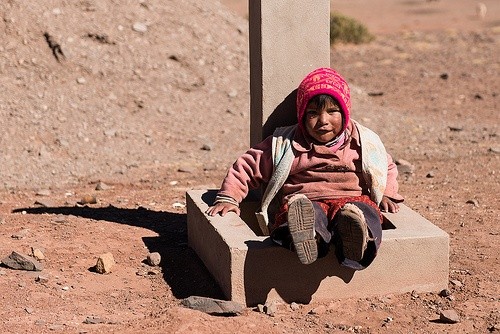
[203,68,405,271]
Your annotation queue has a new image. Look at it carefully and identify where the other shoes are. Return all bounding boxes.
[287,194,318,264]
[338,204,369,261]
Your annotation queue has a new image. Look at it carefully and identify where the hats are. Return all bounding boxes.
[297,68,350,143]
